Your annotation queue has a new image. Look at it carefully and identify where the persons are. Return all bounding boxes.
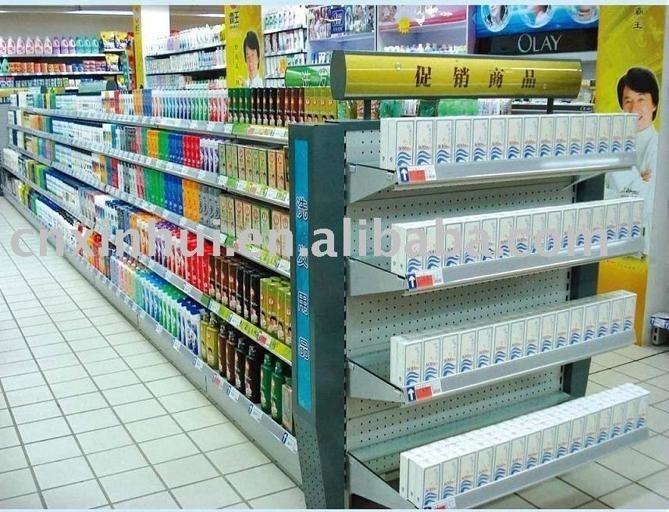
[601,68,660,259]
[239,32,263,87]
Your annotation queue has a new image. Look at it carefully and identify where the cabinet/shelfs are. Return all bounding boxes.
[145,3,305,86]
[0,31,131,102]
[306,5,375,65]
[376,5,468,53]
[341,112,646,510]
[1,87,304,488]
